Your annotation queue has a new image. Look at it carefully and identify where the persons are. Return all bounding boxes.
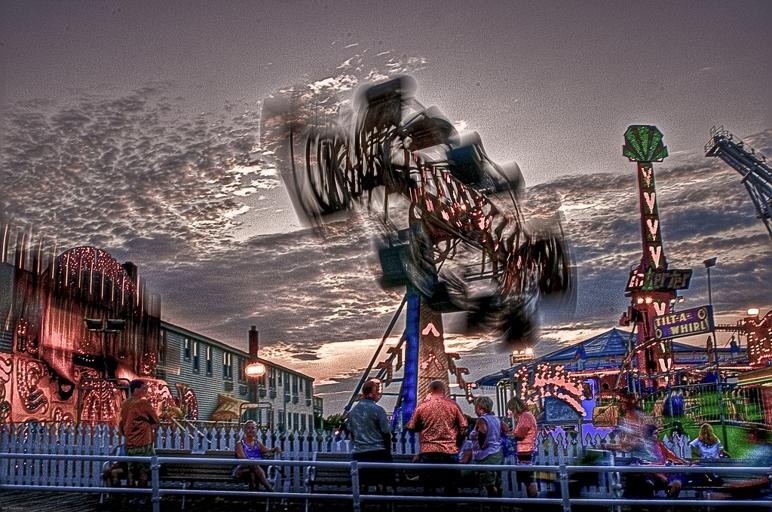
[472,397,509,497]
[508,397,539,498]
[706,335,714,363]
[628,422,699,499]
[730,336,740,356]
[689,423,724,459]
[232,420,281,509]
[459,440,473,464]
[406,381,469,512]
[348,381,395,512]
[581,388,595,424]
[103,379,161,504]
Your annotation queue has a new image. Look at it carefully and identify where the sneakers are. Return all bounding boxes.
[667,480,682,498]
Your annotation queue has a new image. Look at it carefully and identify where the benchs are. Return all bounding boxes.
[99,446,281,512]
[608,455,726,511]
[304,451,422,511]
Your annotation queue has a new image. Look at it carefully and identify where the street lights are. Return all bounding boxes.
[701,257,728,451]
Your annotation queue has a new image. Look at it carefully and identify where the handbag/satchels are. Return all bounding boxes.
[501,437,517,456]
